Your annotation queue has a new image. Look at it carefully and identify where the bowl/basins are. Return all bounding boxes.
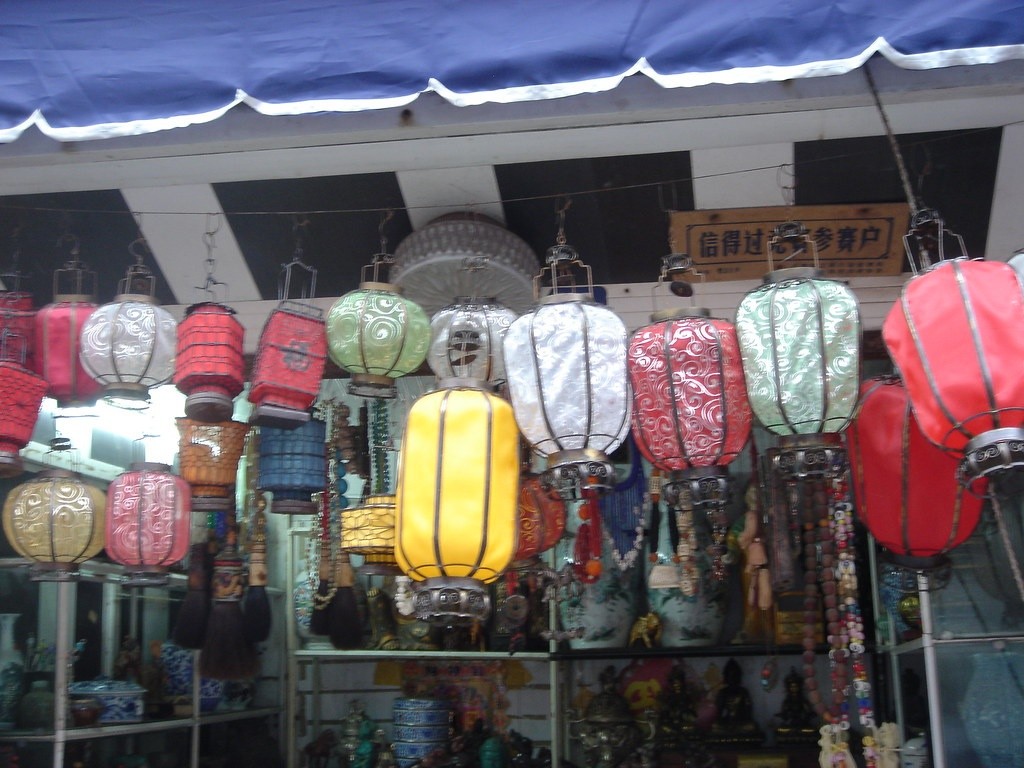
[69,681,148,723]
[393,699,454,768]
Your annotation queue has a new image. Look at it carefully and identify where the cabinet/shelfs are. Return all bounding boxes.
[0,439,286,768]
[866,491,1024,768]
[281,464,876,768]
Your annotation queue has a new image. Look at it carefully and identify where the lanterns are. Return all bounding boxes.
[1,254,1024,624]
